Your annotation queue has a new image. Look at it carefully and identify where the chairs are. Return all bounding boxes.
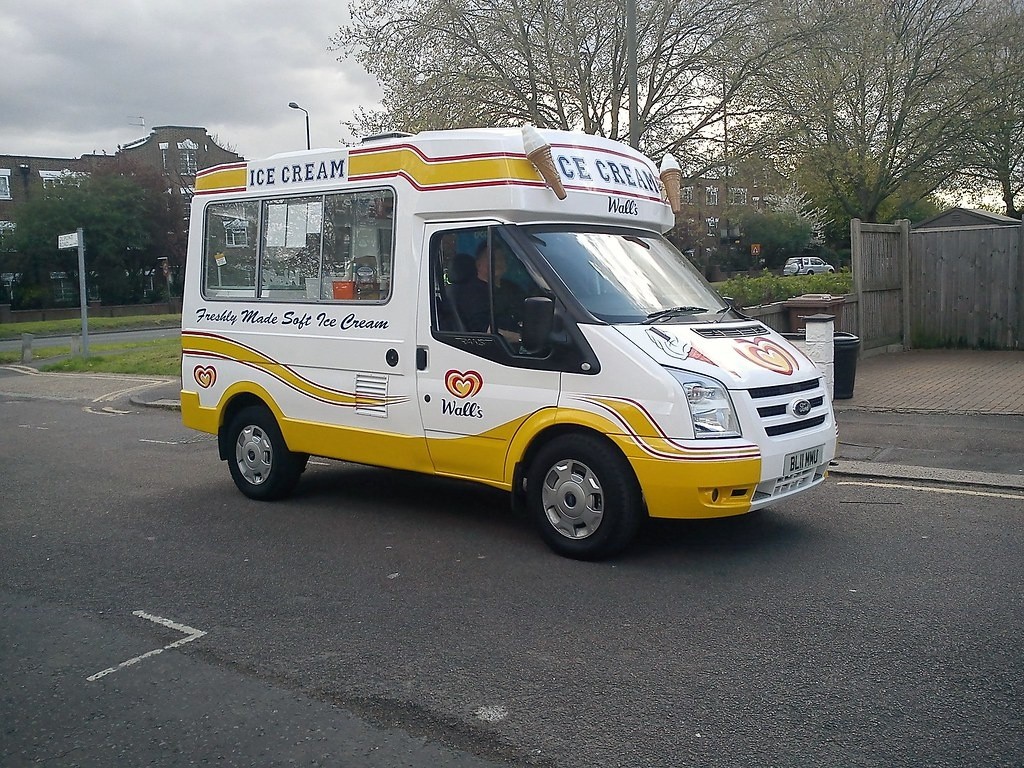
[446,253,488,332]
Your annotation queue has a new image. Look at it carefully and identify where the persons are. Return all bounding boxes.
[455,238,524,353]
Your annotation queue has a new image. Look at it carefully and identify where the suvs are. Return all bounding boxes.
[783,256,835,276]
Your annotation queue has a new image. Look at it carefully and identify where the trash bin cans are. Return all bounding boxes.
[782,293,845,332]
[834,331,861,399]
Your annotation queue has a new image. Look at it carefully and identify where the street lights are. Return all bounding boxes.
[289,102,311,150]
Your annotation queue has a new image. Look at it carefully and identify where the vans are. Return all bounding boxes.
[179,122,839,561]
